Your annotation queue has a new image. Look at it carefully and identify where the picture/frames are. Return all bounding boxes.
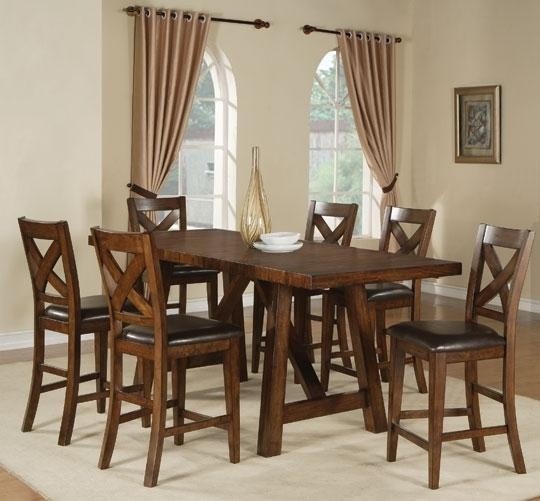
[453,84,502,164]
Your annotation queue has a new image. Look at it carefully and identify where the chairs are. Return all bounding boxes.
[89,225,245,487]
[18,215,153,446]
[126,193,221,322]
[383,221,537,491]
[252,197,360,384]
[316,204,437,395]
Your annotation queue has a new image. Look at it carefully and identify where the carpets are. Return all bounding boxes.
[0,326,538,499]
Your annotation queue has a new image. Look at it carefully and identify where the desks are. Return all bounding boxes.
[88,227,463,459]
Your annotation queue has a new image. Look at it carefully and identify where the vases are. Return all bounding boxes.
[238,146,273,249]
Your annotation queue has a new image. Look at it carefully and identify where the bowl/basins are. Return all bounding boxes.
[260,231,300,244]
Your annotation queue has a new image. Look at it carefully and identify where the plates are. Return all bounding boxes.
[254,239,304,253]
